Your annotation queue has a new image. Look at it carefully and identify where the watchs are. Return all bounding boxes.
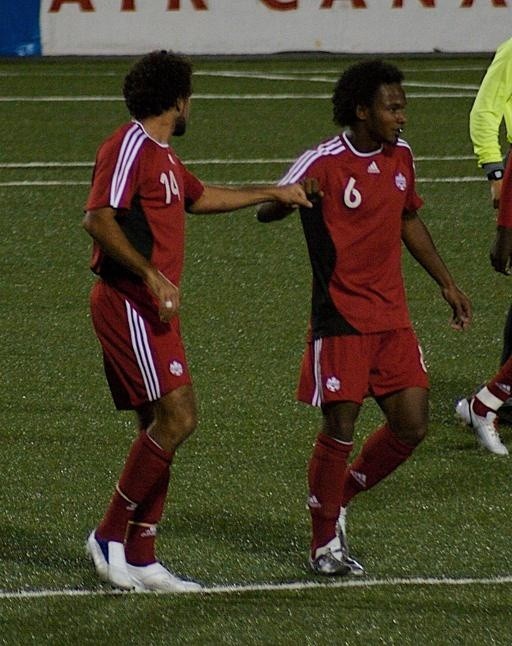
[486,168,505,181]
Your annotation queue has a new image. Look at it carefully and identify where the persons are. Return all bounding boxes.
[448,140,512,460]
[464,34,512,428]
[79,46,325,596]
[254,53,475,581]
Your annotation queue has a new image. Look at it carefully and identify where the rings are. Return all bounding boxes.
[163,299,174,308]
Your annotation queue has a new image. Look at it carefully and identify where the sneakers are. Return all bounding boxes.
[84,527,137,592]
[307,504,366,578]
[124,558,203,593]
[454,395,510,457]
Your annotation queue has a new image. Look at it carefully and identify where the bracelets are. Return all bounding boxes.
[482,162,505,169]
